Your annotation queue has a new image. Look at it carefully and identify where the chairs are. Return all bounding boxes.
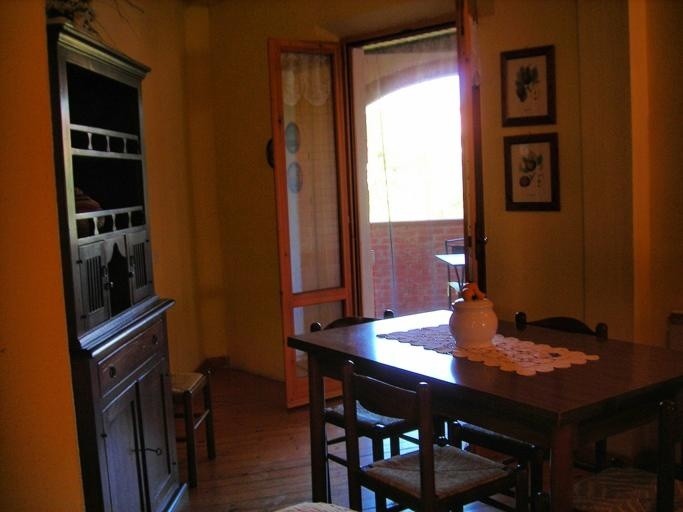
[451,309,611,508]
[171,369,215,488]
[342,358,531,512]
[568,310,683,510]
[312,310,445,512]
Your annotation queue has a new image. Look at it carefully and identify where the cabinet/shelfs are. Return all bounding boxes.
[53,48,157,331]
[96,320,180,510]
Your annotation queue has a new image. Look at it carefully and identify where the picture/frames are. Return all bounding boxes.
[499,44,562,212]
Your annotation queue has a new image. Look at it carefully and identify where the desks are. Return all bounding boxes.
[287,309,682,512]
